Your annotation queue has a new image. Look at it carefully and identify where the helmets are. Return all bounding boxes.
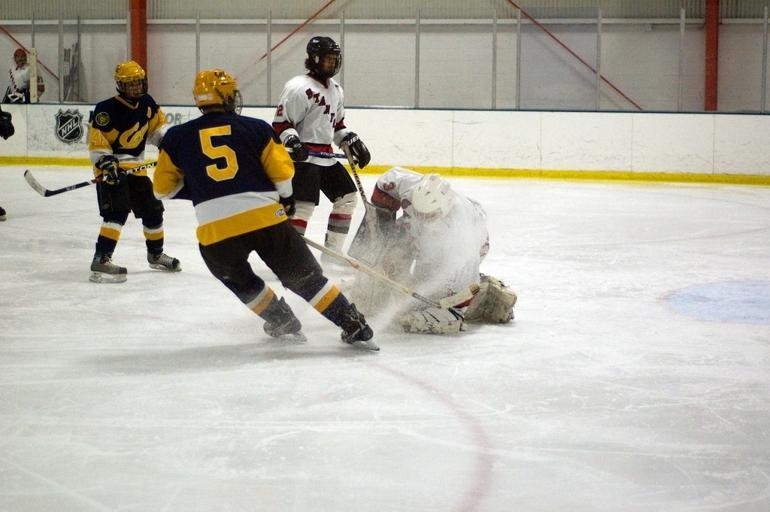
[13,50,27,66]
[194,69,242,118]
[410,172,454,230]
[114,60,148,98]
[304,36,342,77]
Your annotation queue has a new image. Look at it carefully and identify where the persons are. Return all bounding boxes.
[88,60,181,282]
[0,105,16,222]
[271,36,369,264]
[153,71,374,342]
[7,48,44,102]
[351,169,491,333]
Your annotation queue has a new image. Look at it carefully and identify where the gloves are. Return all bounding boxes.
[3,117,14,140]
[288,137,310,160]
[342,133,371,170]
[281,196,297,219]
[100,160,126,184]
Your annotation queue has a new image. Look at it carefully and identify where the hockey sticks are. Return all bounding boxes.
[24,161,157,196]
[300,234,480,308]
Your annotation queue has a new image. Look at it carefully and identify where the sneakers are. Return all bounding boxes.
[340,303,373,344]
[320,246,355,266]
[264,298,301,337]
[148,252,181,269]
[90,255,127,274]
